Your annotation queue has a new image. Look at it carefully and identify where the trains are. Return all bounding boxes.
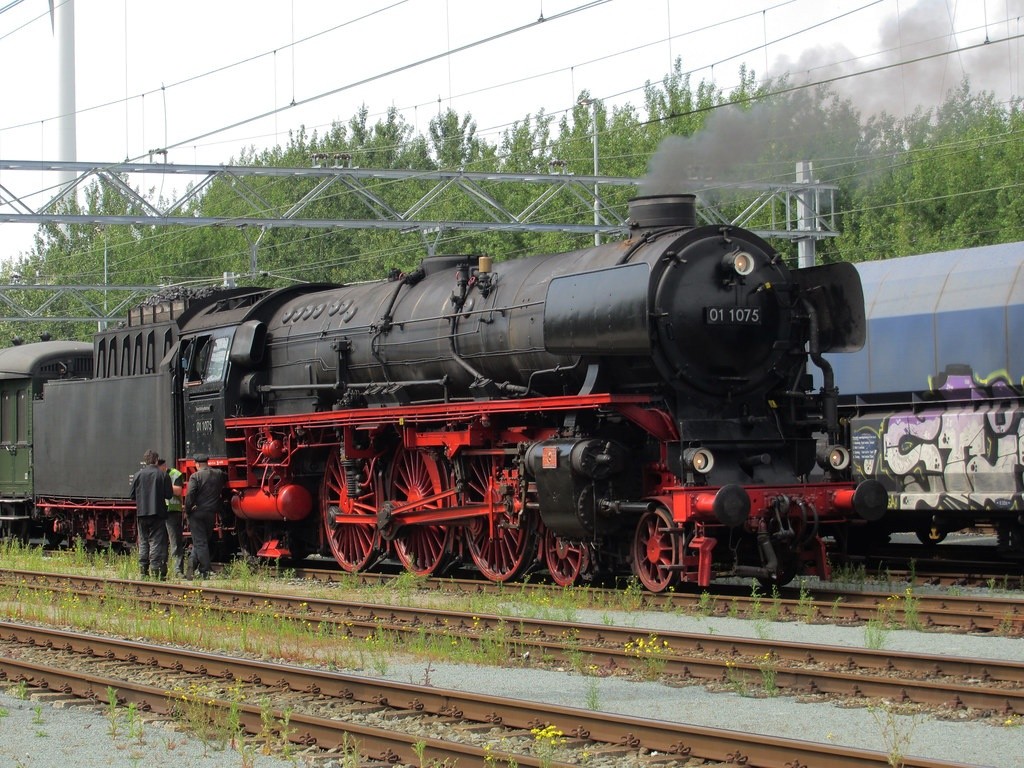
[1,192,892,598]
[795,236,1024,560]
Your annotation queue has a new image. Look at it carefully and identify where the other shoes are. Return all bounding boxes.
[160,572,167,580]
[186,559,195,580]
[175,572,186,579]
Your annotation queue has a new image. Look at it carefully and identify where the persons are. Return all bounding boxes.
[129,449,186,582]
[185,455,232,580]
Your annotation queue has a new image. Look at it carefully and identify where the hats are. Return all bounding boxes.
[158,458,166,466]
[193,453,209,463]
